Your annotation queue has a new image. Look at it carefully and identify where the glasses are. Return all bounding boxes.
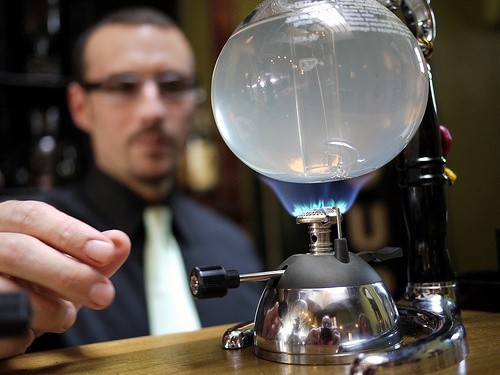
[81,71,203,100]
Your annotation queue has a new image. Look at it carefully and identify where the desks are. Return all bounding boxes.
[0,309,500,374]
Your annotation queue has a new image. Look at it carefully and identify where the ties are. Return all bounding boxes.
[141,205,201,335]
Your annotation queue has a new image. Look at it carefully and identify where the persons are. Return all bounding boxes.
[264,297,374,344]
[0,1,269,362]
